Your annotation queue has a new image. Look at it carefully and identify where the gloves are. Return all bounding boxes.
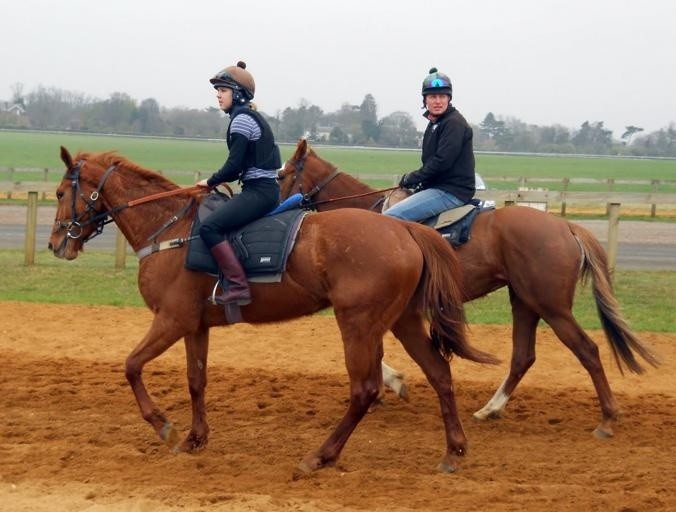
[399,173,419,189]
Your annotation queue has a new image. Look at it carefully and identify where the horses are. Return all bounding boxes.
[276,136,661,439]
[46,144,505,483]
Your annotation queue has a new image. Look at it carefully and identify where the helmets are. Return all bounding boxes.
[422,68,452,104]
[210,61,255,102]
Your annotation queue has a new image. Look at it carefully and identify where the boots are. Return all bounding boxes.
[207,240,253,308]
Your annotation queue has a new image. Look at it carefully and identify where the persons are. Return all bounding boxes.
[195,62,281,306]
[383,68,475,221]
